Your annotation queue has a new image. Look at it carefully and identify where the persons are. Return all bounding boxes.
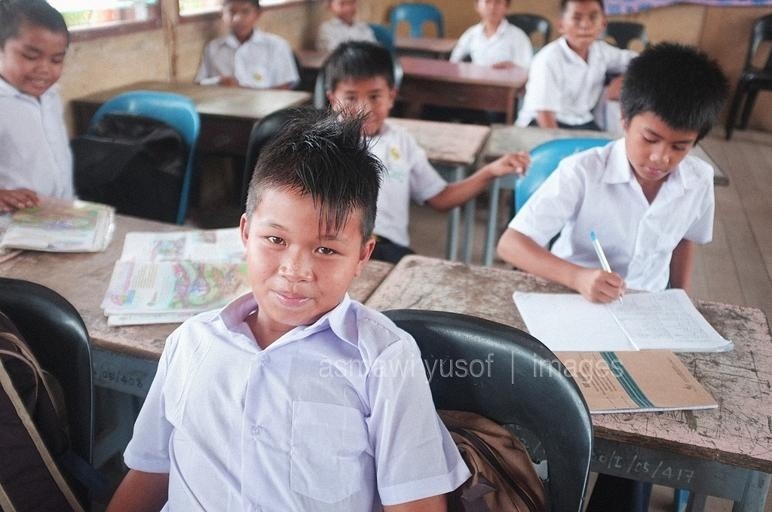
[492,35,732,307]
[102,100,478,512]
[0,0,79,214]
[315,34,534,268]
[315,0,406,92]
[448,0,533,128]
[194,0,302,93]
[514,0,641,131]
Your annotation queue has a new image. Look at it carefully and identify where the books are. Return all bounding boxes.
[98,227,262,328]
[547,348,719,417]
[2,192,116,257]
[513,287,734,360]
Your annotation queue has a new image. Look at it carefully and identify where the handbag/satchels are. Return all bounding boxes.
[70,113,183,224]
[0,311,86,512]
[442,409,547,511]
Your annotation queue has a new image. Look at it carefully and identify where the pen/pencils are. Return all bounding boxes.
[588,229,624,303]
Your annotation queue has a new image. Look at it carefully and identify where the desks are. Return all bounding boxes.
[359,123,772,507]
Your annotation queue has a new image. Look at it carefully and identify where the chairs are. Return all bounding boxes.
[0,4,652,510]
[725,13,772,140]
